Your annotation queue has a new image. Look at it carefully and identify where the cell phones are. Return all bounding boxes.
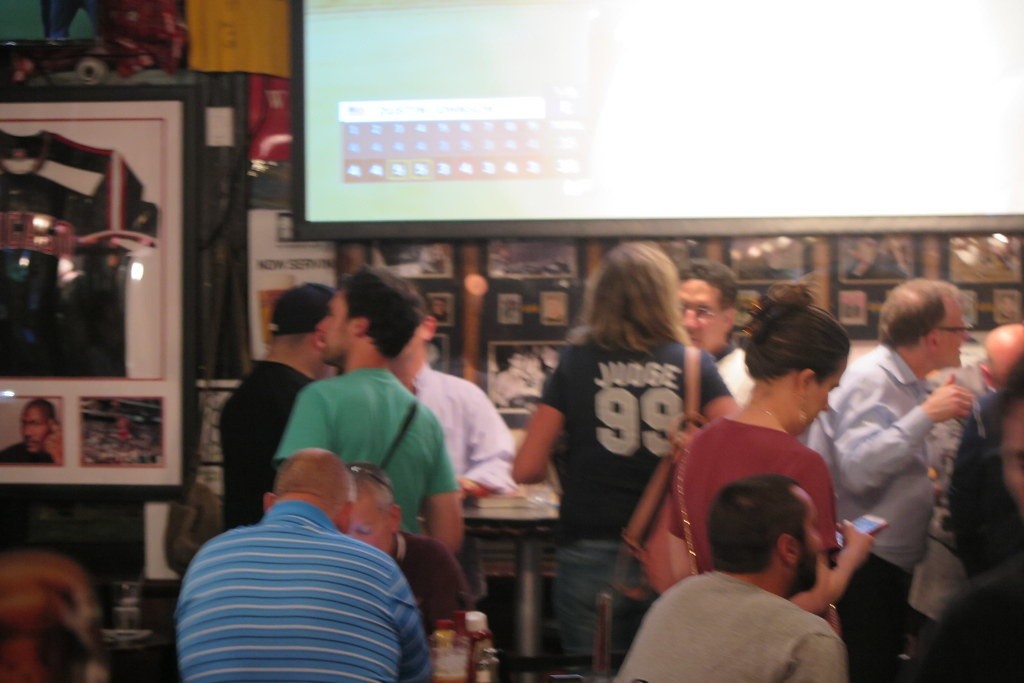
[834,514,888,548]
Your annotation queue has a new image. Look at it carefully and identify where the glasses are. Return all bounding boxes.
[20,413,53,428]
[680,304,718,321]
[937,321,974,338]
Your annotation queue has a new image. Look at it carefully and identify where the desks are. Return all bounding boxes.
[455,496,568,683]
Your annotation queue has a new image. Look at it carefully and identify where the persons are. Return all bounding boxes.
[353,234,1019,410]
[0,399,63,464]
[508,239,1023,683]
[175,265,517,683]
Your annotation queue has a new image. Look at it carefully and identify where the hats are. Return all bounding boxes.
[267,281,340,338]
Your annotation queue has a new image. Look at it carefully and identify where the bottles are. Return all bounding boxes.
[424,611,506,683]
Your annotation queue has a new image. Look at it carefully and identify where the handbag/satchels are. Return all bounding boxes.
[612,346,711,604]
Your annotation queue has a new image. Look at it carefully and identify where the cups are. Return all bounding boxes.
[110,581,145,633]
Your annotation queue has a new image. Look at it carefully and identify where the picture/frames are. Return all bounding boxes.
[0,81,207,503]
